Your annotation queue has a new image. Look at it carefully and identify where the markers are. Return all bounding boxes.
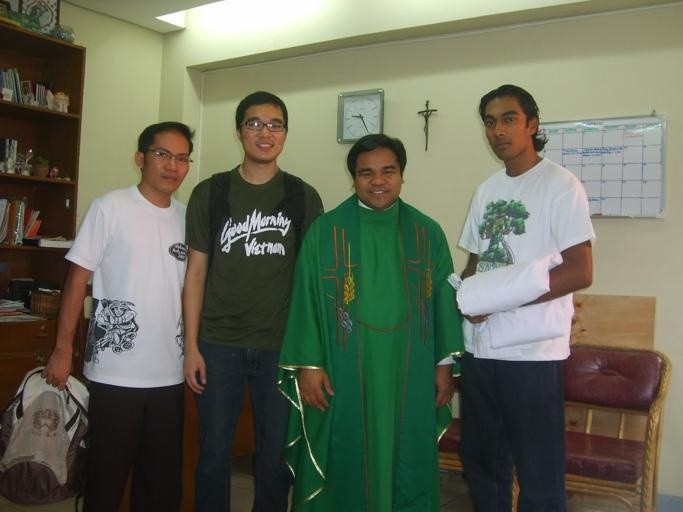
[592,213,602,216]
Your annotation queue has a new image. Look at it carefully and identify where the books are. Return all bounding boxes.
[0,66,74,324]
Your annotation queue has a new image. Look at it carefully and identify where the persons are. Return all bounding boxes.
[183,90,324,512]
[42,121,196,510]
[275,132,464,512]
[446,84,597,510]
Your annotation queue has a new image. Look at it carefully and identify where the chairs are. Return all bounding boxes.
[439,342,672,512]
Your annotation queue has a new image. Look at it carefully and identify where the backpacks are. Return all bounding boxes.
[1,365,92,506]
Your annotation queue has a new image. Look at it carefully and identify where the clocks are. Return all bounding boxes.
[336,87,386,147]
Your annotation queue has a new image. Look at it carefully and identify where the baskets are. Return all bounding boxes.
[30,292,68,320]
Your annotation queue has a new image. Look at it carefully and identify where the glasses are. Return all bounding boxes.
[240,120,287,132]
[148,148,193,167]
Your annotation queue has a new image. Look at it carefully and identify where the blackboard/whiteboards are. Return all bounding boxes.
[536,114,666,219]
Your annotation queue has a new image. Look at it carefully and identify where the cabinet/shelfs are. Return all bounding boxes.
[1,20,87,417]
